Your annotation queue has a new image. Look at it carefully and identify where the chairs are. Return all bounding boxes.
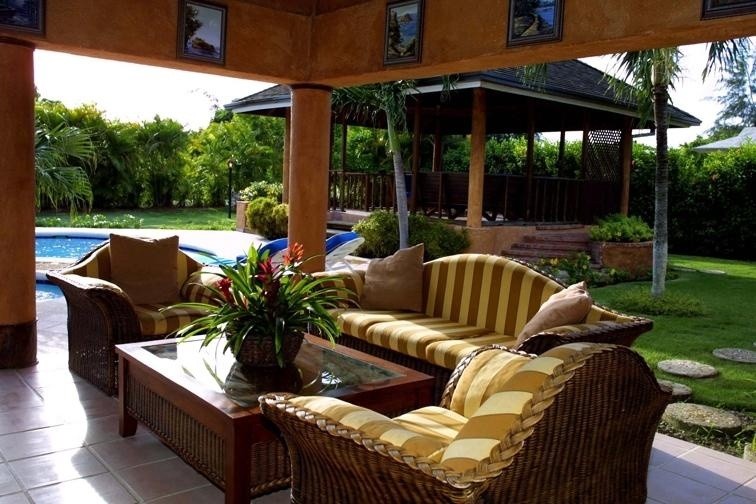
[46,238,246,398]
[257,343,673,504]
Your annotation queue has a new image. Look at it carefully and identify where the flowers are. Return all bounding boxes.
[156,240,362,368]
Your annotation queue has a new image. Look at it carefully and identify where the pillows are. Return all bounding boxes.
[515,281,592,347]
[109,233,181,305]
[360,243,425,312]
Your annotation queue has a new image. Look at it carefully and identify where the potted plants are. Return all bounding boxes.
[588,212,654,278]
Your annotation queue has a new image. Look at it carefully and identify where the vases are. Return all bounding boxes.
[225,332,304,368]
[225,363,302,408]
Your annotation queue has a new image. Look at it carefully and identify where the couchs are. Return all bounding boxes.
[308,254,653,406]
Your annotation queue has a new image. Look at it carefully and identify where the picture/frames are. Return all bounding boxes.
[507,0,565,48]
[699,0,756,21]
[0,0,47,36]
[176,0,228,67]
[383,1,426,66]
